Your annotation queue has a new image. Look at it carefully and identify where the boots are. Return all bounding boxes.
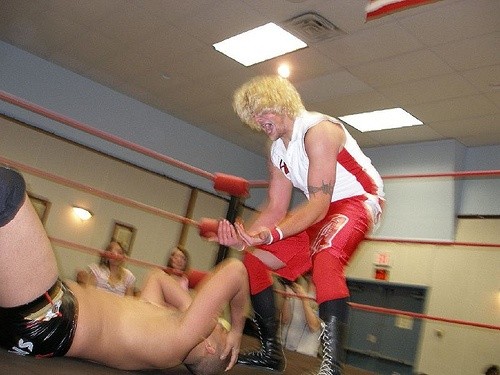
[235,311,286,373]
[315,316,346,375]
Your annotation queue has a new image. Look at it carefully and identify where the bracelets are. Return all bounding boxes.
[238,242,248,252]
[267,227,283,245]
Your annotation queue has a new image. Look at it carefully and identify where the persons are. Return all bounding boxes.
[272,271,322,360]
[0,166,253,375]
[163,247,190,293]
[77,238,135,298]
[206,76,387,375]
